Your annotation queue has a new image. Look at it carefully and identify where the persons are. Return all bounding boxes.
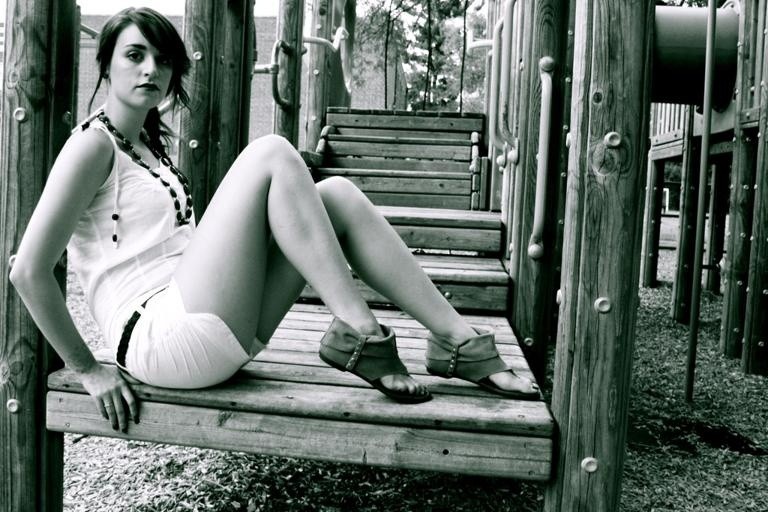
[9,6,536,434]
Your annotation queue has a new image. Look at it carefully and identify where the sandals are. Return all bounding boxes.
[426,324,541,401]
[318,316,432,403]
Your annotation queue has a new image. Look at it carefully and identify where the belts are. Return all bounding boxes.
[116,289,164,372]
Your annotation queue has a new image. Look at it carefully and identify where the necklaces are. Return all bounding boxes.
[82,110,193,227]
